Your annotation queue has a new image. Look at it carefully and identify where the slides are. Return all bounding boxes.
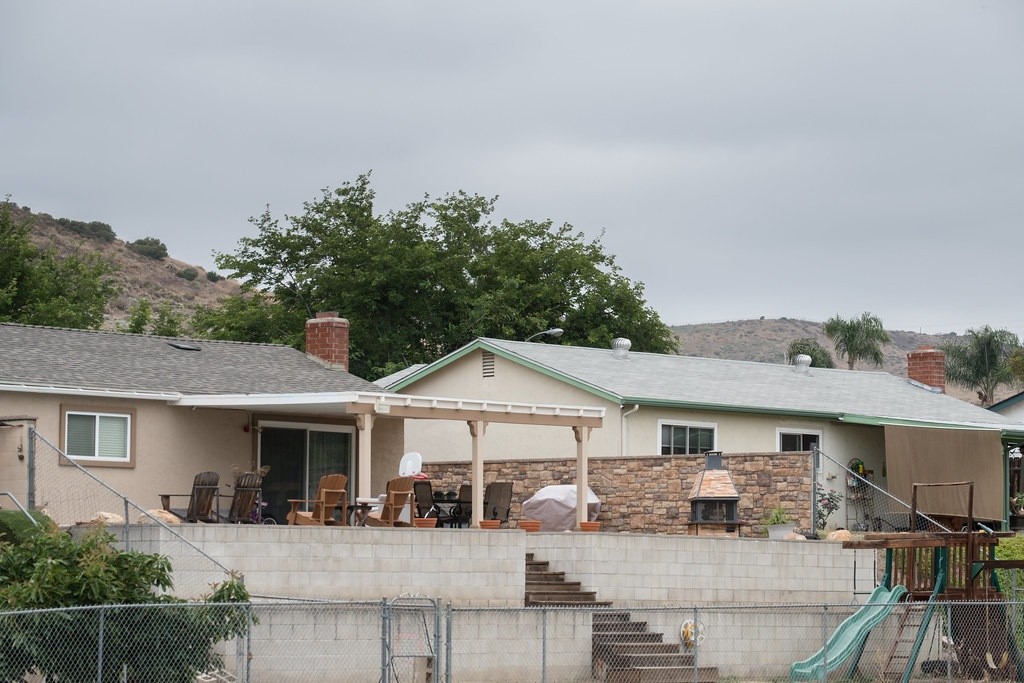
[790,582,909,683]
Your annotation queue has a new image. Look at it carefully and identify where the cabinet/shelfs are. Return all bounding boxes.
[846,458,877,531]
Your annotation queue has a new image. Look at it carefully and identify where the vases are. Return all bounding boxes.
[479,520,501,529]
[518,521,541,532]
[580,522,601,531]
[413,518,437,528]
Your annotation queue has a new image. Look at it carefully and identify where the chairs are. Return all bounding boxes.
[483,486,490,513]
[286,474,348,527]
[157,471,220,523]
[211,473,262,524]
[449,484,472,529]
[469,482,514,524]
[414,481,458,529]
[355,476,416,527]
[355,452,422,504]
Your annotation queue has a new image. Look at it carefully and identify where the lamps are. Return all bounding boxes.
[244,425,263,434]
[524,328,565,342]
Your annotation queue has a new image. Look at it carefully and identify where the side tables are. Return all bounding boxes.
[333,505,377,527]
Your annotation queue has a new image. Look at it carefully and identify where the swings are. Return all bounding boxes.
[984,568,1010,669]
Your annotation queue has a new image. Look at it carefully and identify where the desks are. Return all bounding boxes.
[432,499,488,516]
